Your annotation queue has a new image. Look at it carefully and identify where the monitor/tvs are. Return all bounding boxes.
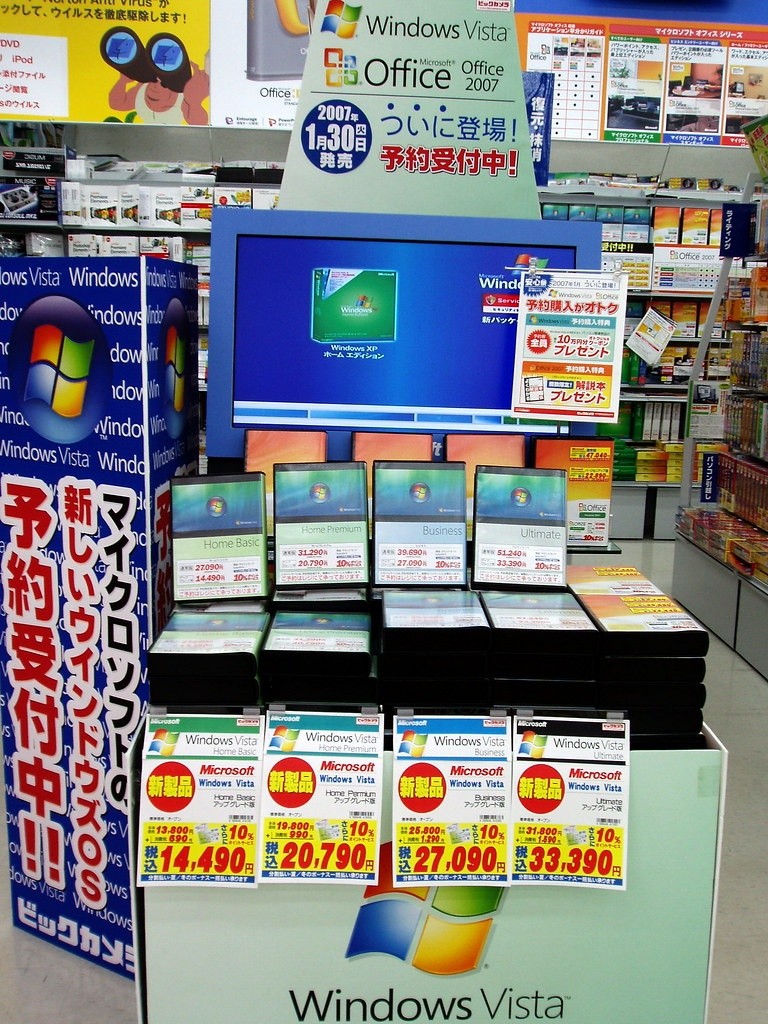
[206,209,602,477]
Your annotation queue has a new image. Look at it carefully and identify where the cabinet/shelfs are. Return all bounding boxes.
[0,150,768,679]
